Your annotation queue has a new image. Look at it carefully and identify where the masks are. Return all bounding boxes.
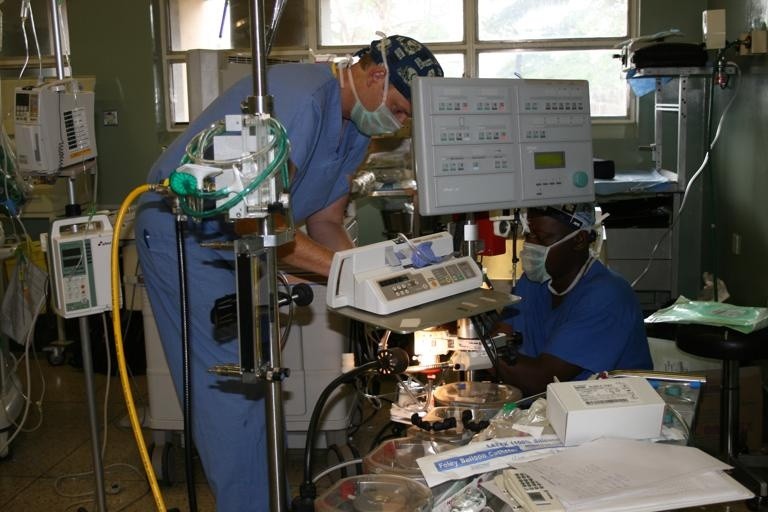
[521,240,549,285]
[350,103,400,141]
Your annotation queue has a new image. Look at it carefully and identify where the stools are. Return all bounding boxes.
[673,324,768,512]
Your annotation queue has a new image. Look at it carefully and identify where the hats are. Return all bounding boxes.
[371,35,443,102]
[533,202,596,238]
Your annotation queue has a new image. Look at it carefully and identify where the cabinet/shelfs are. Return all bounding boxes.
[595,191,681,311]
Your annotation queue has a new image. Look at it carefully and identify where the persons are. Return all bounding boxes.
[136,31,444,512]
[434,200,653,411]
[644,293,767,335]
[478,438,755,512]
